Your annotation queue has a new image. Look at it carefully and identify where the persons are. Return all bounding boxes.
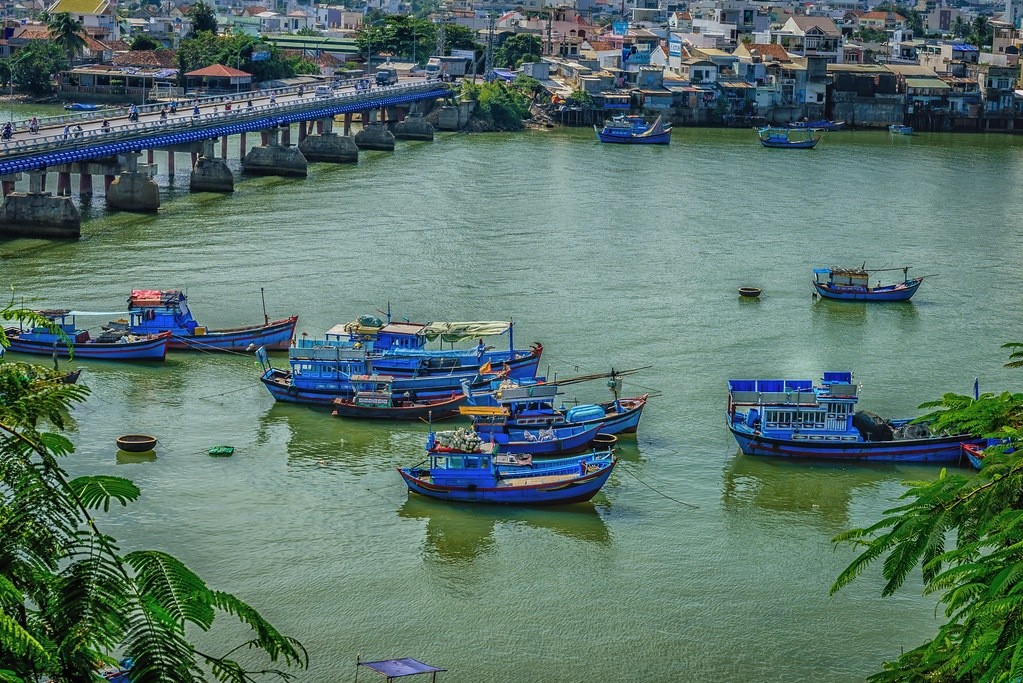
[103,118,110,127]
[150,82,159,92]
[129,104,138,117]
[299,84,304,93]
[77,123,83,131]
[194,105,199,114]
[270,92,277,103]
[248,99,253,106]
[64,124,71,139]
[225,97,232,108]
[170,100,176,115]
[1,121,12,133]
[237,105,241,108]
[213,104,218,112]
[437,67,445,82]
[161,109,167,118]
[30,117,37,133]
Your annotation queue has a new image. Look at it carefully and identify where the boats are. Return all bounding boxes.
[593,112,673,144]
[256,301,650,507]
[100,287,300,352]
[724,371,1015,473]
[888,124,913,135]
[758,126,821,149]
[64,103,103,111]
[89,657,135,683]
[811,260,940,301]
[789,116,846,132]
[0,309,172,361]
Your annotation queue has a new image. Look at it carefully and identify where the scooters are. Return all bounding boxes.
[128,112,139,123]
[101,122,110,133]
[29,124,39,135]
[1,123,13,142]
[297,89,303,97]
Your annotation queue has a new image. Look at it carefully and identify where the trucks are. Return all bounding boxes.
[375,68,398,86]
[425,55,467,81]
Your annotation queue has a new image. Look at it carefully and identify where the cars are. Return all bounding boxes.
[315,86,334,98]
[357,78,371,89]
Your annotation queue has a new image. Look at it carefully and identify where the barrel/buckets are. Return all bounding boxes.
[746,409,758,427]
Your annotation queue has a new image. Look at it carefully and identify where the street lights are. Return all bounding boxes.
[10,51,33,121]
[315,36,331,84]
[237,40,254,93]
